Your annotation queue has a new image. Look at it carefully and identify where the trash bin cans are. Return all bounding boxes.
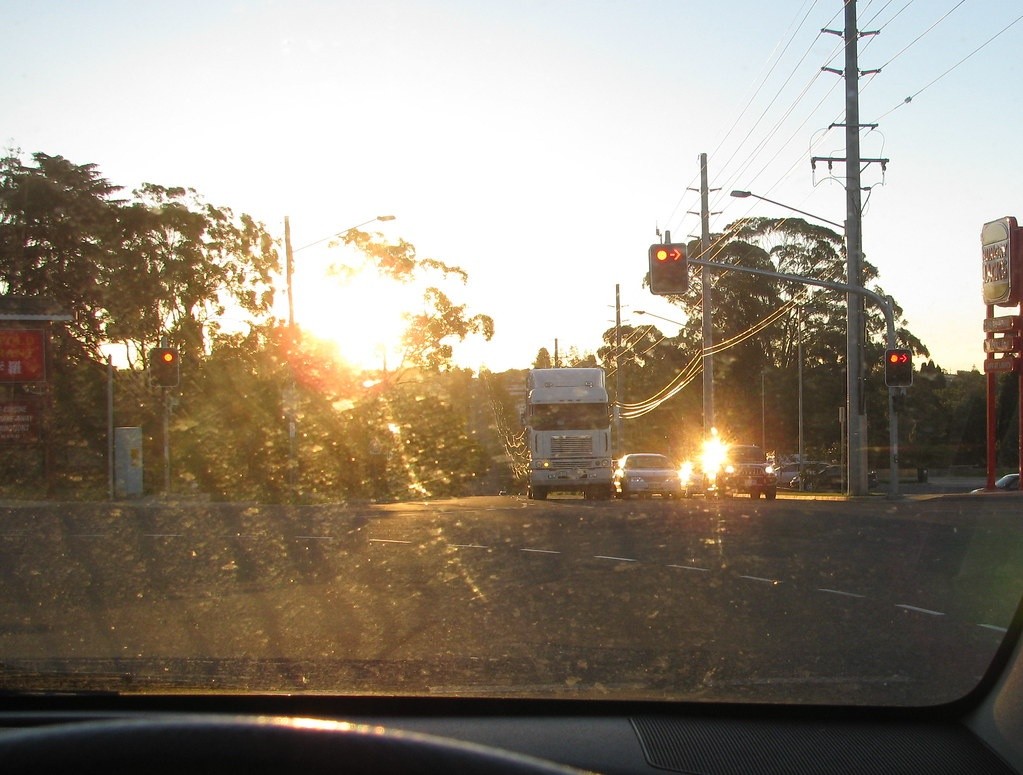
[917,464,928,483]
[114,427,143,499]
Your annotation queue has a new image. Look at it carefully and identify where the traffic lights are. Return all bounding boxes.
[149,347,180,387]
[885,348,912,387]
[650,242,689,295]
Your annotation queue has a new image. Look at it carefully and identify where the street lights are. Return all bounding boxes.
[731,190,869,496]
[634,309,714,441]
[282,213,397,340]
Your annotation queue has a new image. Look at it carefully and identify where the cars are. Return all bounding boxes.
[612,444,884,503]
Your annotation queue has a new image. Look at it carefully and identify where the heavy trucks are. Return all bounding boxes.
[523,365,611,500]
